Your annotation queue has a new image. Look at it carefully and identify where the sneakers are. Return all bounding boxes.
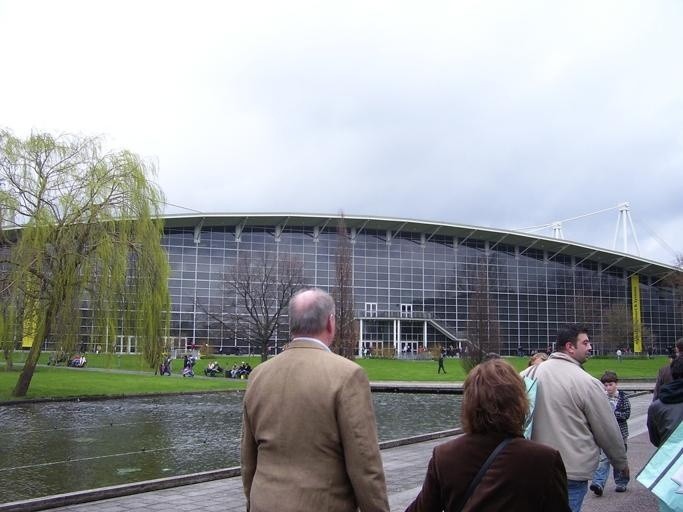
[590,481,603,496]
[614,483,627,492]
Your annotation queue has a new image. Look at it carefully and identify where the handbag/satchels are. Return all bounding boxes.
[635,420,683,512]
[522,364,537,440]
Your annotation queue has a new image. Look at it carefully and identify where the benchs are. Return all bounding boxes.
[201,369,248,379]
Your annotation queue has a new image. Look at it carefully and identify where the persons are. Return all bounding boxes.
[241,287,390,512]
[80,355,86,367]
[647,339,683,511]
[230,362,250,378]
[159,357,171,376]
[404,359,573,512]
[617,348,622,363]
[204,361,221,377]
[182,355,193,377]
[438,354,447,374]
[519,325,631,512]
